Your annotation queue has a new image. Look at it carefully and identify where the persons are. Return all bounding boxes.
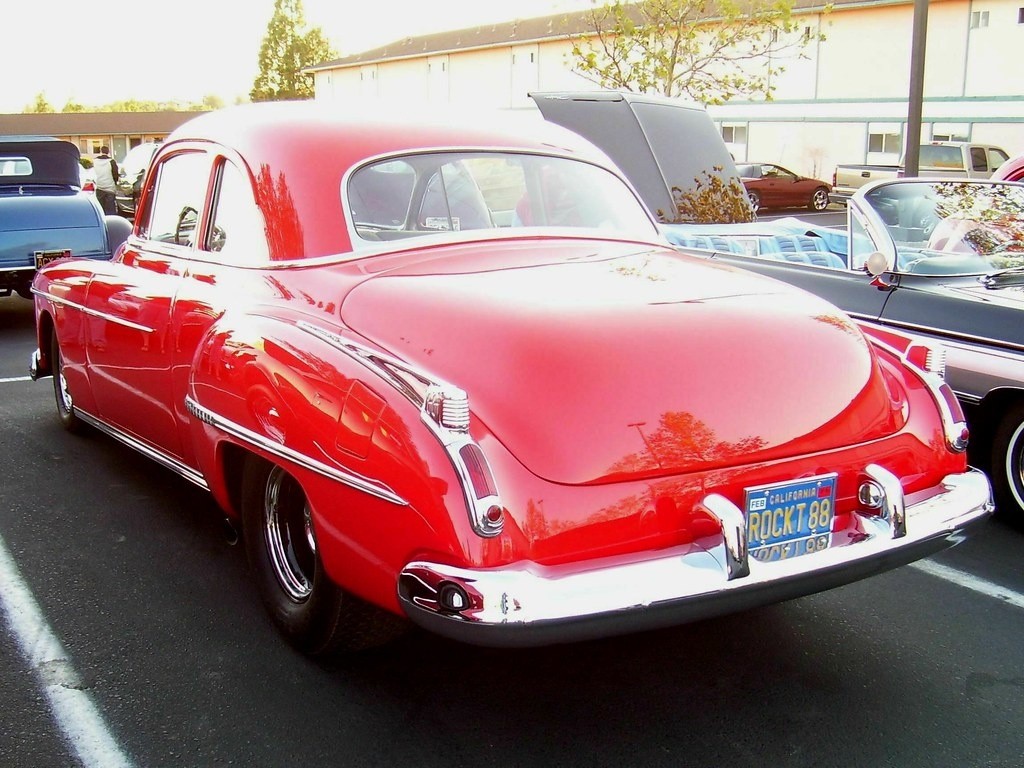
[131,169,145,212]
[93,146,118,215]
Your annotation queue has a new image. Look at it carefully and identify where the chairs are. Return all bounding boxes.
[759,249,846,269]
[674,235,745,255]
[759,234,829,254]
[896,252,928,271]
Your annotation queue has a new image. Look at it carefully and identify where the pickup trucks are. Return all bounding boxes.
[524,91,1024,532]
[828,141,1011,230]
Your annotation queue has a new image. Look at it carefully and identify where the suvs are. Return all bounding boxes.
[29,100,996,667]
[110,142,160,229]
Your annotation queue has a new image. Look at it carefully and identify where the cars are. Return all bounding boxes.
[928,156,1024,264]
[0,134,134,300]
[733,162,832,214]
